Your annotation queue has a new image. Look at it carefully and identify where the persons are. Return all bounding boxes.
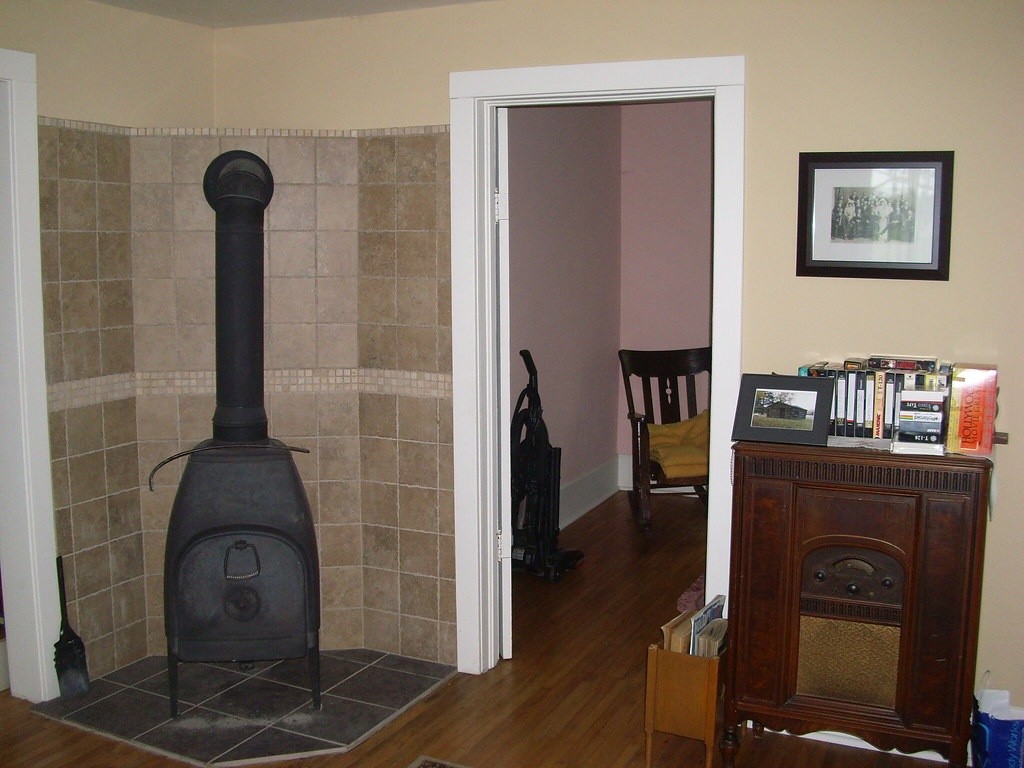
[831,190,914,242]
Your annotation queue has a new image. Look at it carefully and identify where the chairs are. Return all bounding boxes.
[617,347,709,534]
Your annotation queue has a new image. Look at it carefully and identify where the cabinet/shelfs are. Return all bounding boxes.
[719,443,993,768]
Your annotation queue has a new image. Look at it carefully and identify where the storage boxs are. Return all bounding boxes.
[644,644,724,768]
[946,362,998,458]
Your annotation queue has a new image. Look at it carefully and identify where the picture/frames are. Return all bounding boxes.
[731,373,834,447]
[795,150,954,280]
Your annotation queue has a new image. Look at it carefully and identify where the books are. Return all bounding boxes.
[798,355,952,457]
[660,594,728,657]
